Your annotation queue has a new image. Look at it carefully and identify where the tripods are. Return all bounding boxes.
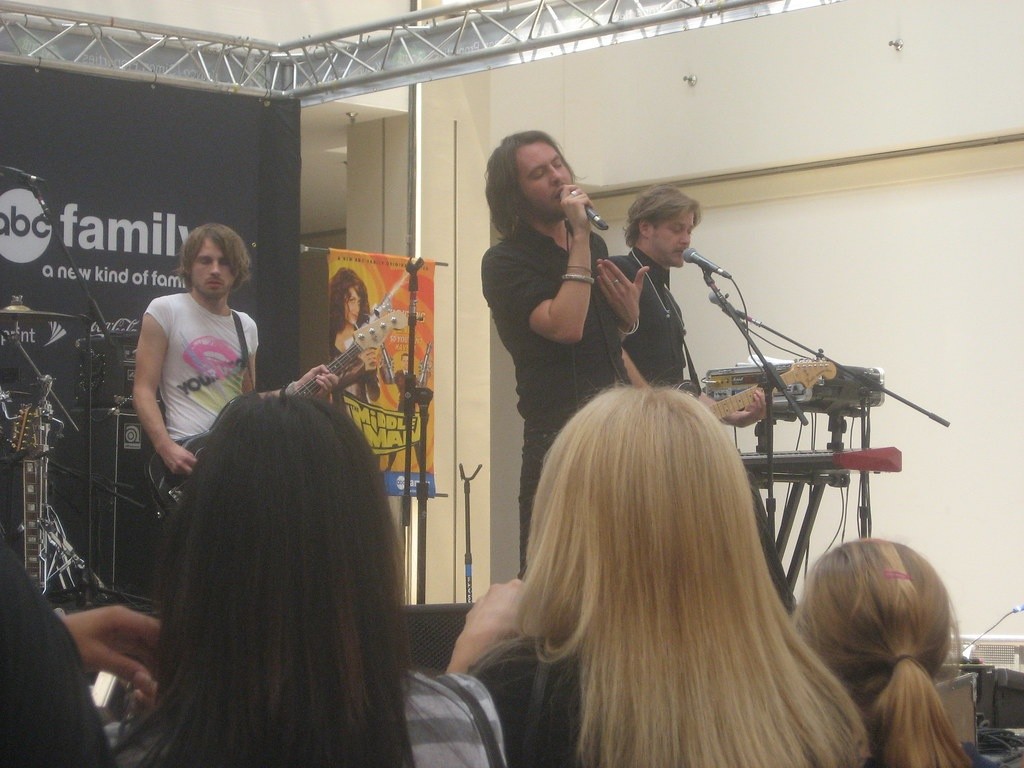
[18,179,164,617]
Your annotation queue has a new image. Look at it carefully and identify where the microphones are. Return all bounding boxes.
[584,205,608,231]
[682,248,732,279]
[0,165,44,183]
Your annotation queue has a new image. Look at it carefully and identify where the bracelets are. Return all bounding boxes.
[618,317,640,336]
[566,263,594,277]
[279,384,288,396]
[561,273,595,285]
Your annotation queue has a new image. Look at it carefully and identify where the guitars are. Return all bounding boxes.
[145,307,408,525]
[707,357,837,421]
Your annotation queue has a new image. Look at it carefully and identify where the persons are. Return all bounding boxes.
[444,381,869,768]
[330,267,381,404]
[100,389,507,768]
[0,538,167,768]
[607,184,765,428]
[790,535,1001,768]
[479,130,652,578]
[384,352,423,472]
[132,221,339,476]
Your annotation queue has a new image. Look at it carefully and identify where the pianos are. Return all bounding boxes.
[739,447,903,474]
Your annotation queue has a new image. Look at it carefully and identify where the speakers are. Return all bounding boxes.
[408,602,475,673]
[70,410,165,602]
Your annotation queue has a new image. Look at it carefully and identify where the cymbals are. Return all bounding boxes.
[0,305,82,324]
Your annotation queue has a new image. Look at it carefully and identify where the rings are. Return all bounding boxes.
[613,279,619,284]
[571,191,577,196]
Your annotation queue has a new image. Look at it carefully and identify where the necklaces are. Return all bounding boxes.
[631,250,671,319]
